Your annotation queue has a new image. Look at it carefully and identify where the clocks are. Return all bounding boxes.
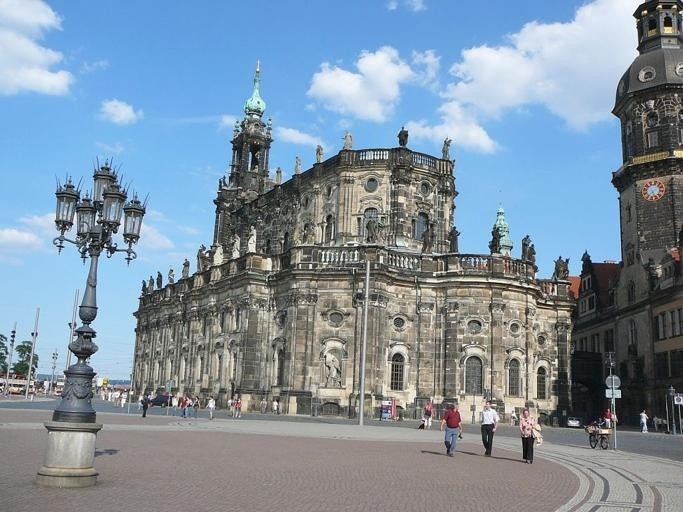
[642,180,665,201]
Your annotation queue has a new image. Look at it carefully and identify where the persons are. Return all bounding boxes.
[422,400,434,430]
[603,408,612,429]
[639,409,649,433]
[510,410,518,426]
[440,405,462,457]
[219,127,452,213]
[43,378,279,418]
[479,400,498,457]
[141,216,592,298]
[519,409,536,464]
[611,410,618,428]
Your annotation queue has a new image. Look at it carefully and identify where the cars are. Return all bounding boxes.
[567,416,582,428]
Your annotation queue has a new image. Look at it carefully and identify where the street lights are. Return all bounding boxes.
[33,155,151,491]
[48,348,59,395]
[668,384,678,435]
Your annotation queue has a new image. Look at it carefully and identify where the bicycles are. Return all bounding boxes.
[584,420,610,450]
